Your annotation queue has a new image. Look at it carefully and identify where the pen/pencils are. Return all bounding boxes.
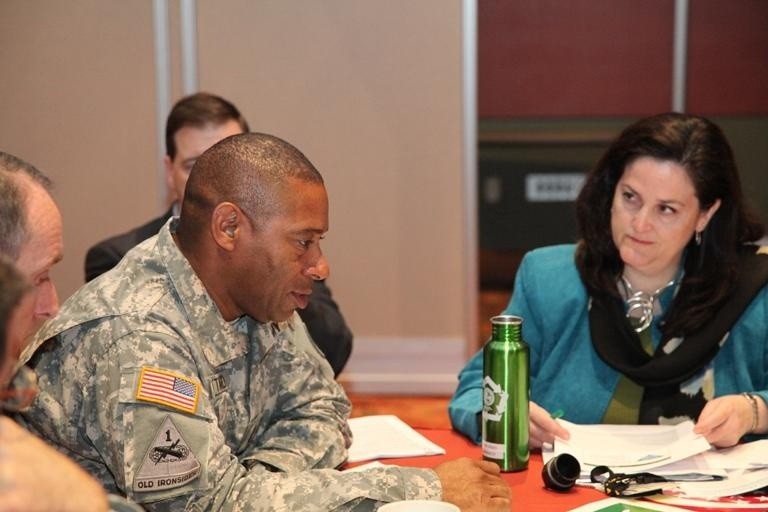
[551,409,564,420]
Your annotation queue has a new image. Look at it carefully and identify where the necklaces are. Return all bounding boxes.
[617,274,676,333]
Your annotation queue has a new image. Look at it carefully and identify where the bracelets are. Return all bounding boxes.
[740,391,761,437]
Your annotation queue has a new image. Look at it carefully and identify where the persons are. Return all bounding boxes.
[85,90,353,389]
[3,131,512,511]
[0,152,109,509]
[447,111,768,454]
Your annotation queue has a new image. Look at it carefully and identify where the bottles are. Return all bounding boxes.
[483,315,529,472]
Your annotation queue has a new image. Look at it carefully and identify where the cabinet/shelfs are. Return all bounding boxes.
[477,2,768,119]
[0,0,477,400]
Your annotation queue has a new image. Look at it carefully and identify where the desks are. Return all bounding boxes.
[350,426,767,511]
